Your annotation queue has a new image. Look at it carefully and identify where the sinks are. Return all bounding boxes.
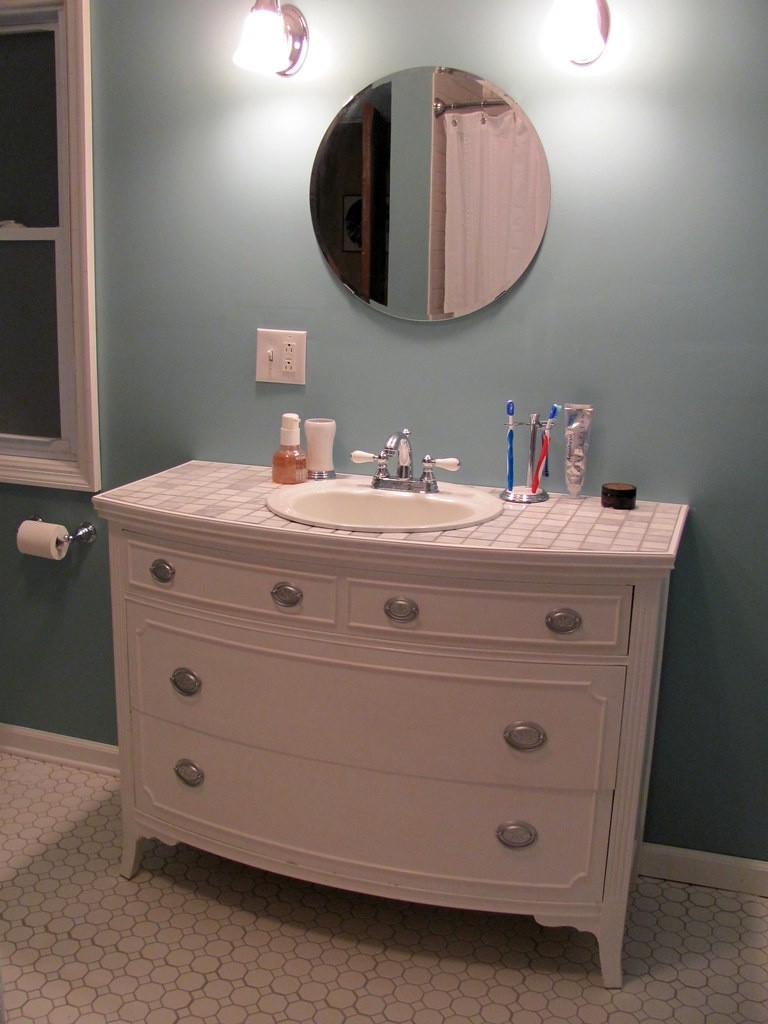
[265,489,504,532]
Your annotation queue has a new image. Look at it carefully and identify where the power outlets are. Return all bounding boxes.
[256,328,306,385]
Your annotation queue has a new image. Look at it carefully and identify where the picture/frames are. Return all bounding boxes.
[341,194,363,253]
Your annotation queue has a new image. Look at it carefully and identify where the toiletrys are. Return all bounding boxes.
[271,412,308,484]
[563,402,596,497]
[601,482,637,511]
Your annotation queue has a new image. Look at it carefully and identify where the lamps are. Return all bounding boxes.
[233,0,310,77]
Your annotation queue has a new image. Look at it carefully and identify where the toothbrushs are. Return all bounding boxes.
[531,403,562,493]
[506,400,515,492]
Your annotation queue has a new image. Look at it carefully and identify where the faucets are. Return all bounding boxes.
[383,427,413,480]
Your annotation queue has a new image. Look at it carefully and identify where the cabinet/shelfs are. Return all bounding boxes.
[104,520,675,987]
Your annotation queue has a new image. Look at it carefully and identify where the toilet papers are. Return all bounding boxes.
[16,519,70,561]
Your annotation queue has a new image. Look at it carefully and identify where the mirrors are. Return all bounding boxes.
[310,67,551,321]
